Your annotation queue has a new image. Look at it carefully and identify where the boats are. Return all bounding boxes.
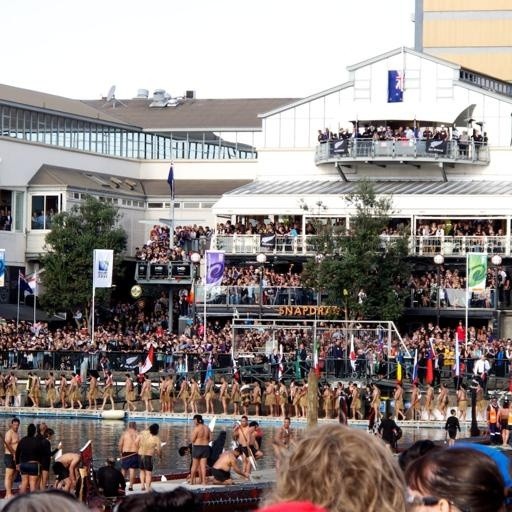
[125,471,213,483]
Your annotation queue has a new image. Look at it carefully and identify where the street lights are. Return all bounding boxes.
[314,253,326,308]
[189,250,201,326]
[254,253,267,324]
[491,254,504,334]
[434,253,446,327]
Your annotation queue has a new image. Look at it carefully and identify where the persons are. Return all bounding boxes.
[178,415,263,486]
[0,216,512,421]
[257,397,512,512]
[4,418,208,512]
[318,124,487,162]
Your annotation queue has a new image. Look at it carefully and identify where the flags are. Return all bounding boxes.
[166,166,176,201]
[387,69,405,103]
